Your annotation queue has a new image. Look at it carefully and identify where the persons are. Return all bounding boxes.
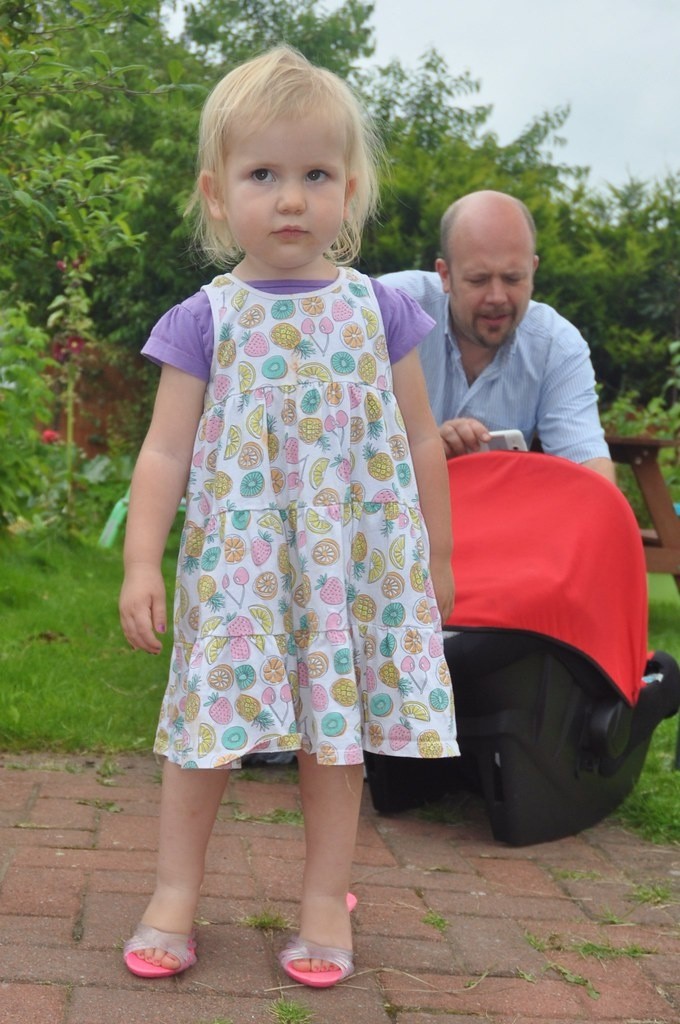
[119,47,460,989]
[374,190,616,488]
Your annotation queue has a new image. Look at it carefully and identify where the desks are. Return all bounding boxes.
[604,434,680,593]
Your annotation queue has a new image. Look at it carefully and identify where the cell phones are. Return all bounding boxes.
[478,428,529,451]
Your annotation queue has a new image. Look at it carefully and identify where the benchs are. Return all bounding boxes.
[640,528,658,545]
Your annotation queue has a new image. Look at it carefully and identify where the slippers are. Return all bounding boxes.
[278,892,356,986]
[122,920,197,977]
